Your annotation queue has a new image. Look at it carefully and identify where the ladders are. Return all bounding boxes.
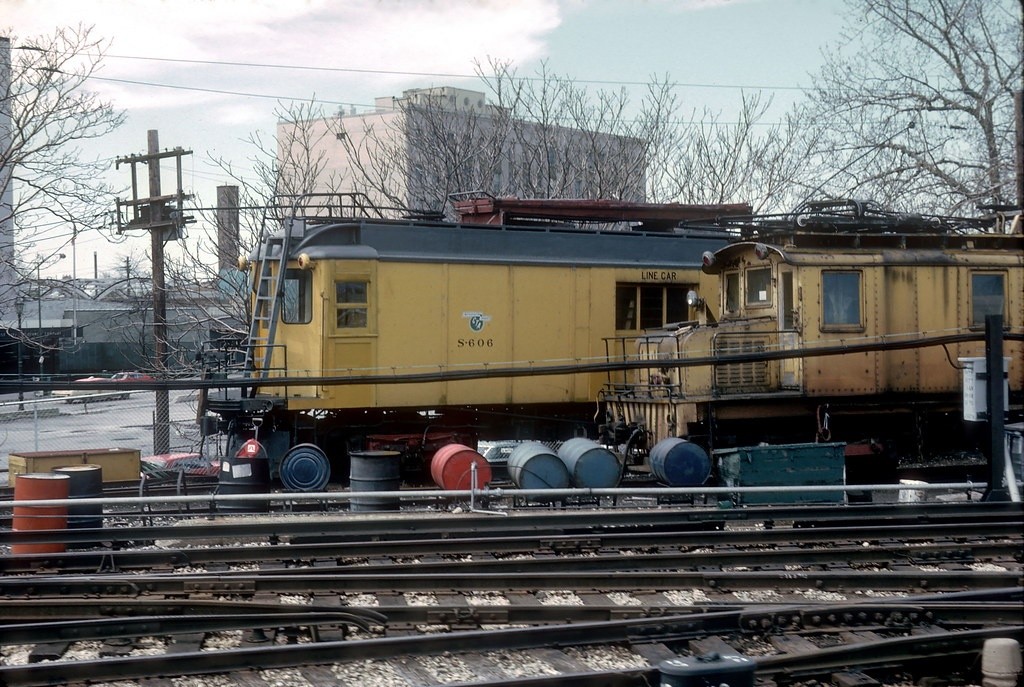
[244,236,288,377]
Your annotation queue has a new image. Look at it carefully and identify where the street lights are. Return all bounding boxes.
[14,301,28,411]
[36,250,67,383]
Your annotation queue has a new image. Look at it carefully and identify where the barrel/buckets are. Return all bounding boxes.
[219,458,271,512]
[431,443,492,490]
[50,463,103,549]
[507,442,570,502]
[350,451,401,512]
[649,439,711,485]
[12,473,70,553]
[280,443,331,491]
[558,437,621,488]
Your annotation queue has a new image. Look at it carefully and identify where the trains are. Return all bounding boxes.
[603,195,1024,470]
[197,190,754,480]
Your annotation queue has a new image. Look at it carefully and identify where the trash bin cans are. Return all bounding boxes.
[712,440,848,507]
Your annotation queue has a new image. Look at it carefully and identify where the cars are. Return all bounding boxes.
[110,371,147,380]
